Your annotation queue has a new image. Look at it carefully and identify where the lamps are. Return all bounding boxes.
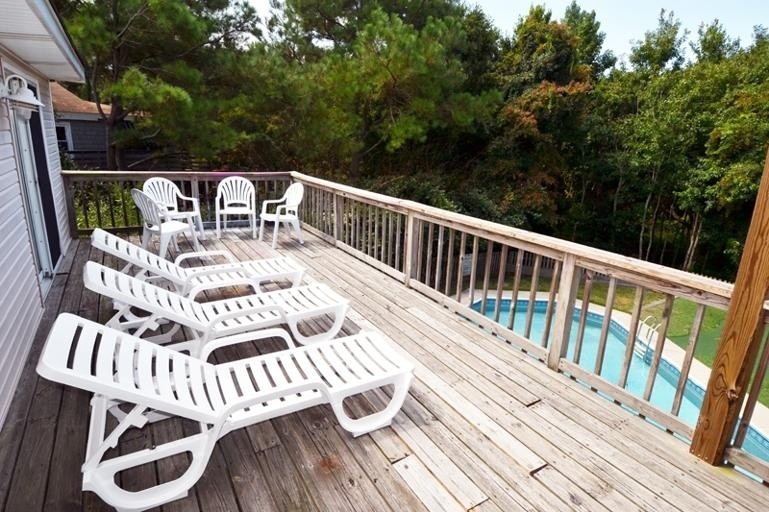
[0,75,46,121]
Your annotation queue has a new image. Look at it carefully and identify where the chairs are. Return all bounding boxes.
[90,228,309,326]
[258,182,304,249]
[83,260,352,432]
[143,177,206,243]
[215,176,257,239]
[36,311,416,512]
[131,187,200,260]
